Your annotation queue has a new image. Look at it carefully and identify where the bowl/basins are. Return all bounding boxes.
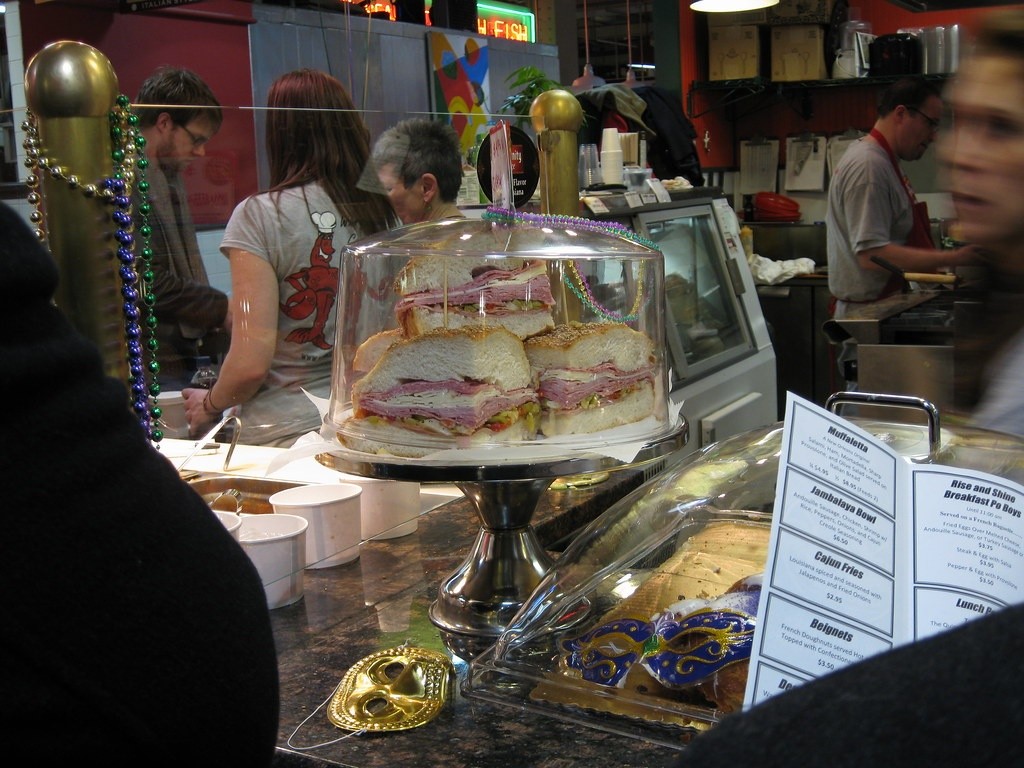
[339,472,421,540]
[234,515,309,611]
[621,168,653,193]
[268,482,364,568]
[751,192,801,223]
[211,509,244,548]
[148,390,188,440]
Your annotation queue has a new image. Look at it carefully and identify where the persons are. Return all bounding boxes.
[943,12,1024,433]
[129,65,468,447]
[827,77,973,348]
[0,199,281,768]
[670,601,1024,768]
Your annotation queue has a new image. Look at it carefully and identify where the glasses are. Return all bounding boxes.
[906,105,940,134]
[175,119,211,148]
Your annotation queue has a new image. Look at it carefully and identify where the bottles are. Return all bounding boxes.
[188,355,225,440]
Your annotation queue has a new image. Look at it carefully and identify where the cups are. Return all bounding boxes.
[578,127,624,188]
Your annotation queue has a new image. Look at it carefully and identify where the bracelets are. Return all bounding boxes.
[208,387,224,411]
[203,391,222,421]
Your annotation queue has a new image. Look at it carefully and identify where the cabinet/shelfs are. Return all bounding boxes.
[756,285,846,423]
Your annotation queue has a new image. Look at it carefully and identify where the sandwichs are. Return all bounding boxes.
[336,223,656,457]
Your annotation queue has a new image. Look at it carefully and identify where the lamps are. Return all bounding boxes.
[572,0,606,86]
[624,0,650,88]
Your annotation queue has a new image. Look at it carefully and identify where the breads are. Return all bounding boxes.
[556,521,770,715]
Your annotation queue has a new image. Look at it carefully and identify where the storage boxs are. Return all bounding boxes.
[707,0,836,81]
